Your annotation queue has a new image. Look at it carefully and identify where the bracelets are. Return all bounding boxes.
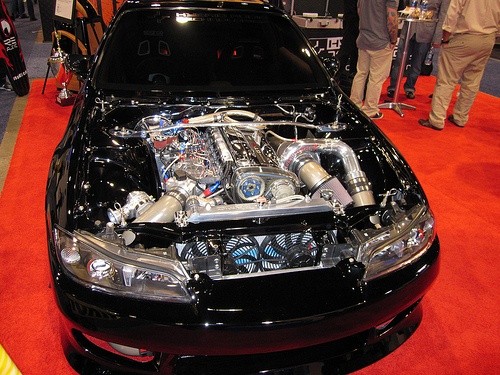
[441,39,449,44]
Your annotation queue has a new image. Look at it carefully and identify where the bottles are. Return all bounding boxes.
[408,0,417,20]
[419,0,429,20]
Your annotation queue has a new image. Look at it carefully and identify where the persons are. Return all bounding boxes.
[351,0,398,119]
[8,0,37,22]
[418,0,500,130]
[387,0,452,98]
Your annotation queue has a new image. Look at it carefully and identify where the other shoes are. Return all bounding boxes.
[428,94,433,98]
[406,91,414,99]
[449,114,464,127]
[370,112,383,119]
[387,90,394,98]
[419,118,443,131]
[0,82,13,91]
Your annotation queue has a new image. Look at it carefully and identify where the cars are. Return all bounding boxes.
[44,0,440,375]
